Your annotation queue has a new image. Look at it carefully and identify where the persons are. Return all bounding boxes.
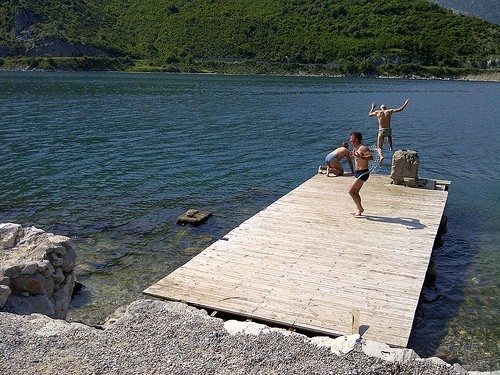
[317,142,354,177]
[368,98,410,164]
[349,132,374,216]
